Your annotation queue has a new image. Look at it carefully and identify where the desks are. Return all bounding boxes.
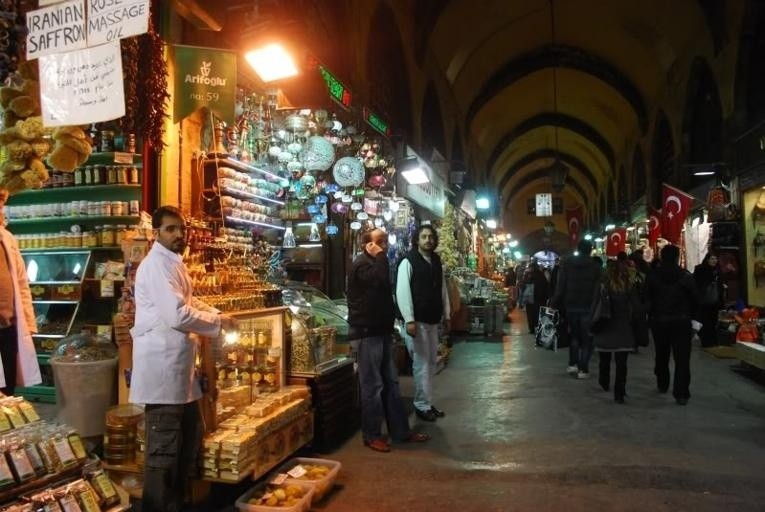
[735,340,765,371]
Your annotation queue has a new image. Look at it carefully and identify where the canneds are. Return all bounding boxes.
[1,130,144,247]
[216,330,277,386]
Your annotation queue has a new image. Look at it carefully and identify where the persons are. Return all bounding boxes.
[507,240,723,407]
[1,185,45,397]
[345,228,430,450]
[128,206,241,512]
[394,224,453,422]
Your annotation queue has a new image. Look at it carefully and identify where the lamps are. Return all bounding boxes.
[543,2,573,193]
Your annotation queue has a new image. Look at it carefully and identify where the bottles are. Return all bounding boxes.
[40,164,140,186]
[214,327,281,391]
[127,134,136,154]
[14,225,139,251]
[4,200,138,219]
[100,130,114,153]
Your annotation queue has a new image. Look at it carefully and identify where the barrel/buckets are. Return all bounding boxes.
[47,355,118,437]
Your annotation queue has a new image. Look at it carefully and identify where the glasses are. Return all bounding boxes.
[157,224,187,233]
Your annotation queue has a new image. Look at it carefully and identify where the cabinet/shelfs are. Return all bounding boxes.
[709,219,740,252]
[116,303,289,434]
[14,250,122,403]
[2,149,142,252]
[268,278,353,374]
[294,222,323,248]
[200,156,294,251]
[753,211,765,288]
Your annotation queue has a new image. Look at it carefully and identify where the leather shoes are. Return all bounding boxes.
[402,431,431,442]
[415,408,436,422]
[431,405,444,417]
[363,438,390,452]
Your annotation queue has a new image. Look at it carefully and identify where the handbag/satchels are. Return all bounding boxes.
[592,278,612,321]
[535,305,561,352]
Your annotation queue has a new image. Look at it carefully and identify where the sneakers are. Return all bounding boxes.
[566,364,579,373]
[676,397,690,405]
[578,369,590,379]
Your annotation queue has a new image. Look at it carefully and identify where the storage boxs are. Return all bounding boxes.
[273,456,343,502]
[235,478,313,512]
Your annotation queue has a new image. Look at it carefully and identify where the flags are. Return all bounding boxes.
[660,182,695,245]
[605,229,627,256]
[565,205,583,248]
[647,203,662,248]
